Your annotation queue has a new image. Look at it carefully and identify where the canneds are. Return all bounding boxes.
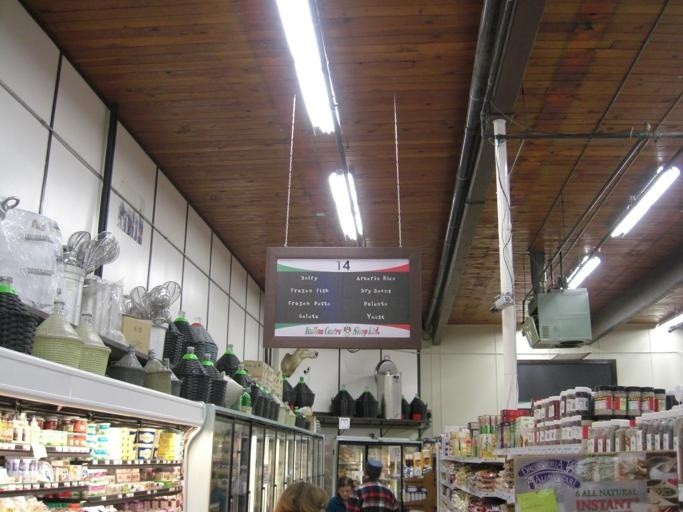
[30,417,86,447]
[534,385,683,452]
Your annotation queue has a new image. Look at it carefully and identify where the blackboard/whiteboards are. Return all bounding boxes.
[266,247,422,350]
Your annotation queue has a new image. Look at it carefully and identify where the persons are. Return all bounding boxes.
[272,481,331,511]
[322,476,355,511]
[344,456,400,512]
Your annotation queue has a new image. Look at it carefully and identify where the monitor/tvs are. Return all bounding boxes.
[516,359,617,405]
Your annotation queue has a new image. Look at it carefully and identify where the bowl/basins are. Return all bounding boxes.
[650,462,678,480]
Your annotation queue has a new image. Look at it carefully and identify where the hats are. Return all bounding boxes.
[365,459,382,473]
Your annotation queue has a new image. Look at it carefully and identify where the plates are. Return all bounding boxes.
[650,484,679,499]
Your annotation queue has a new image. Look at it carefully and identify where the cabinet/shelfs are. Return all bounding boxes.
[0,346,208,510]
[187,403,325,512]
[438,444,681,511]
[333,436,425,511]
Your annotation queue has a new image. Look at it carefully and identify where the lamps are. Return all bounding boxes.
[609,165,681,239]
[276,0,342,139]
[329,168,365,246]
[565,254,601,290]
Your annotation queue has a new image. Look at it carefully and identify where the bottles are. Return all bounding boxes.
[534,386,683,452]
